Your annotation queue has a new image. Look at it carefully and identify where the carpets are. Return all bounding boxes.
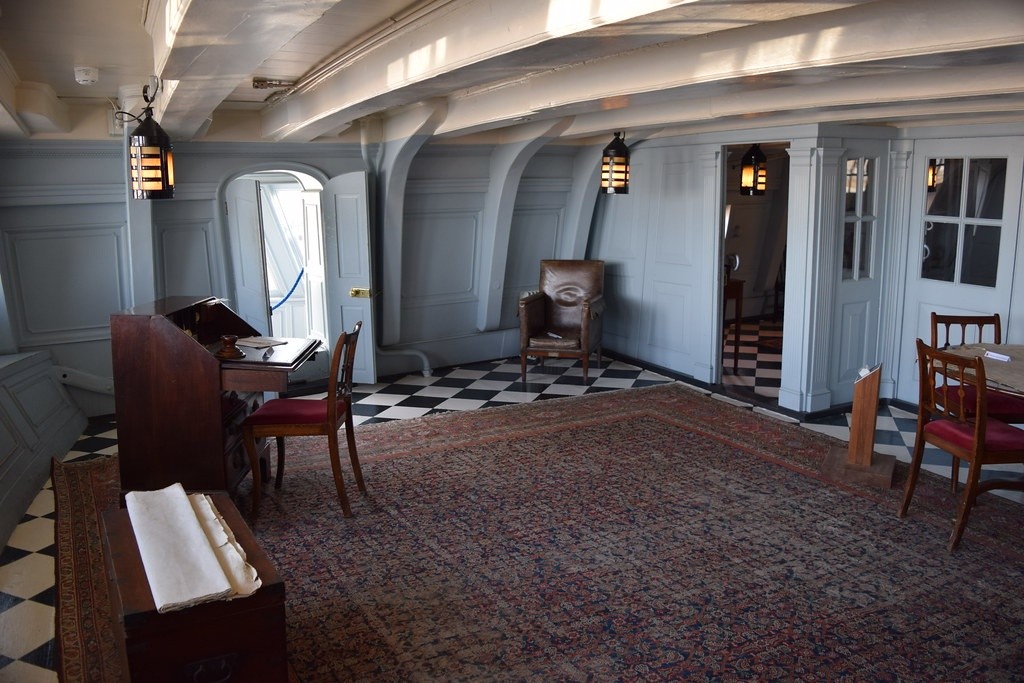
[50,379,1024,683]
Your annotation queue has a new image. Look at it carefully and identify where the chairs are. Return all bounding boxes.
[930,312,1024,495]
[896,338,1024,551]
[239,320,366,517]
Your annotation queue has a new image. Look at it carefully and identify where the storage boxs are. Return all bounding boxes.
[102,492,290,683]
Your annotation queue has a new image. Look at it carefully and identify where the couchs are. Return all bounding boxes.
[519,258,604,385]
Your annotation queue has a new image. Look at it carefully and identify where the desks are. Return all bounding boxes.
[926,343,1024,401]
[721,279,748,369]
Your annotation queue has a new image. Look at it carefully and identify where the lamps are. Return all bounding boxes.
[602,131,630,194]
[739,144,767,196]
[927,159,937,192]
[129,106,174,199]
